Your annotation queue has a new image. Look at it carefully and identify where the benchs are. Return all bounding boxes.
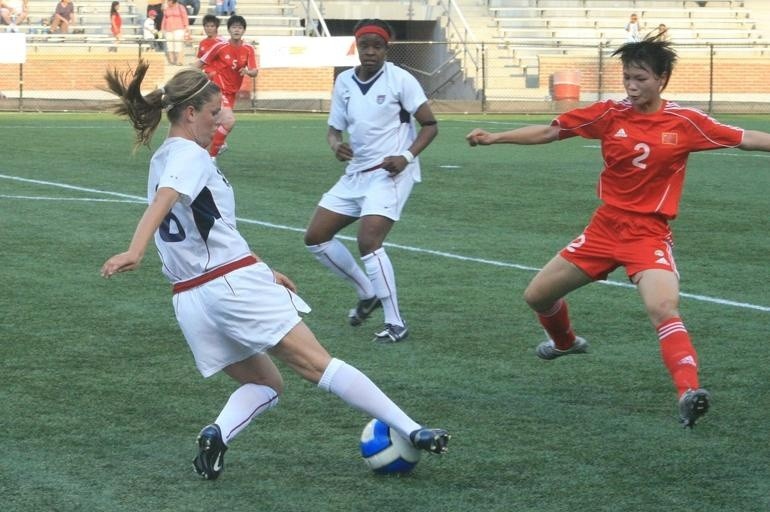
[1,2,316,52]
[481,1,770,91]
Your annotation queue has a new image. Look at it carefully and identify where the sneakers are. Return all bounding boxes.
[678,388,712,430]
[193,424,227,480]
[348,294,409,343]
[535,336,589,360]
[410,428,452,454]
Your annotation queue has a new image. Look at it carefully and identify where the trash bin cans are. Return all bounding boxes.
[553,69,582,100]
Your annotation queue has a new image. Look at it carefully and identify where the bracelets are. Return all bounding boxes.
[401,147,415,164]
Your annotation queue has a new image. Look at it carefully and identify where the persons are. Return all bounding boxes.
[98,60,450,481]
[192,15,224,75]
[657,24,669,43]
[49,0,76,36]
[464,40,769,426]
[143,9,159,51]
[0,1,29,33]
[110,2,122,47]
[159,0,189,66]
[217,0,237,17]
[302,20,441,343]
[185,15,259,160]
[625,13,641,42]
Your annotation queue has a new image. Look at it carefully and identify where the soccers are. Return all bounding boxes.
[359,416,420,477]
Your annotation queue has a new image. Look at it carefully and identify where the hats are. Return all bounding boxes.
[148,10,158,16]
